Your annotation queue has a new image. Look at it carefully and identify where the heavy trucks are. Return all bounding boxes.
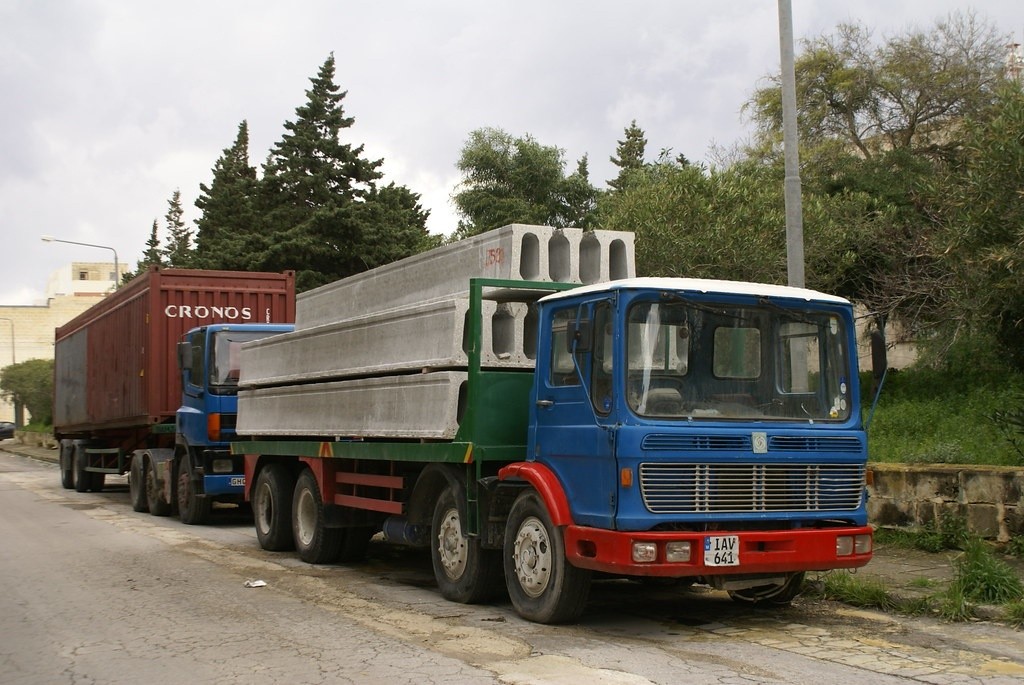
[52,270,296,526]
[229,269,889,624]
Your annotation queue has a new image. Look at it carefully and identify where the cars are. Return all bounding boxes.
[0,421,16,440]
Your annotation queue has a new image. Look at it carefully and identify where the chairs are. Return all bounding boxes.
[712,392,755,411]
[638,388,680,414]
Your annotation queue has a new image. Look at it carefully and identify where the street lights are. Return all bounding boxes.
[42,235,119,294]
